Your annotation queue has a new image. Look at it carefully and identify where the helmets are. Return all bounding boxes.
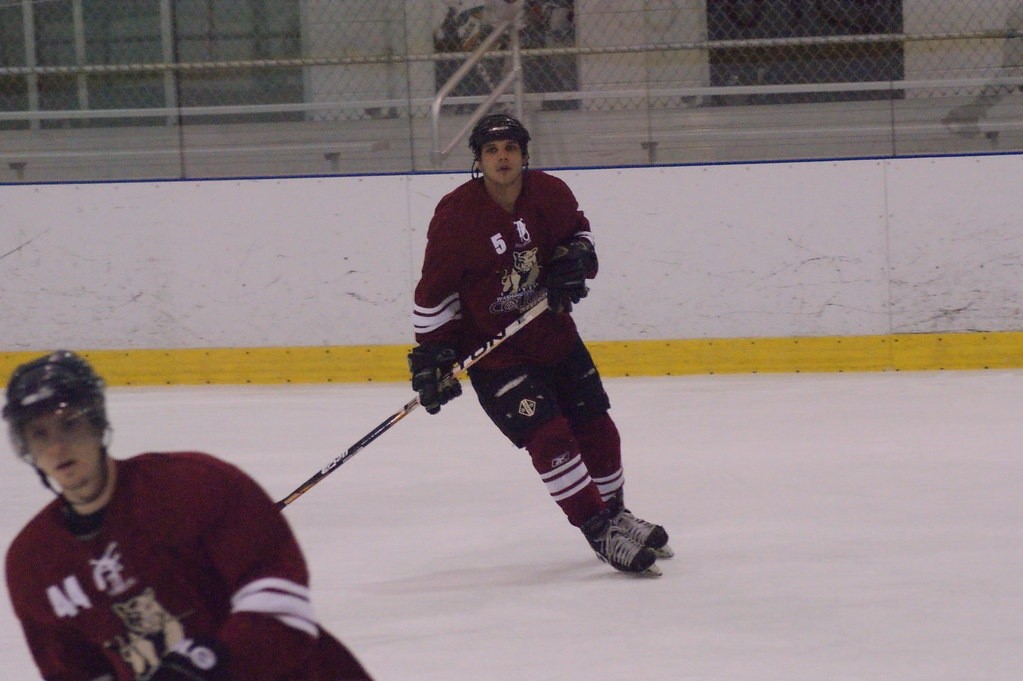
[468,112,529,158]
[3,350,106,428]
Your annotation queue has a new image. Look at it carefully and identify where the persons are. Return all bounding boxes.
[0,350,372,681]
[407,113,674,580]
[434,0,582,113]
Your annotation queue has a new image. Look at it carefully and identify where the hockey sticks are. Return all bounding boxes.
[272,279,578,513]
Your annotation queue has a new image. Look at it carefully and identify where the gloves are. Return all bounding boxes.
[545,247,588,318]
[146,633,230,681]
[408,345,462,413]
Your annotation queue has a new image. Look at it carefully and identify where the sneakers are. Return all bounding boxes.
[590,519,663,576]
[612,508,673,557]
